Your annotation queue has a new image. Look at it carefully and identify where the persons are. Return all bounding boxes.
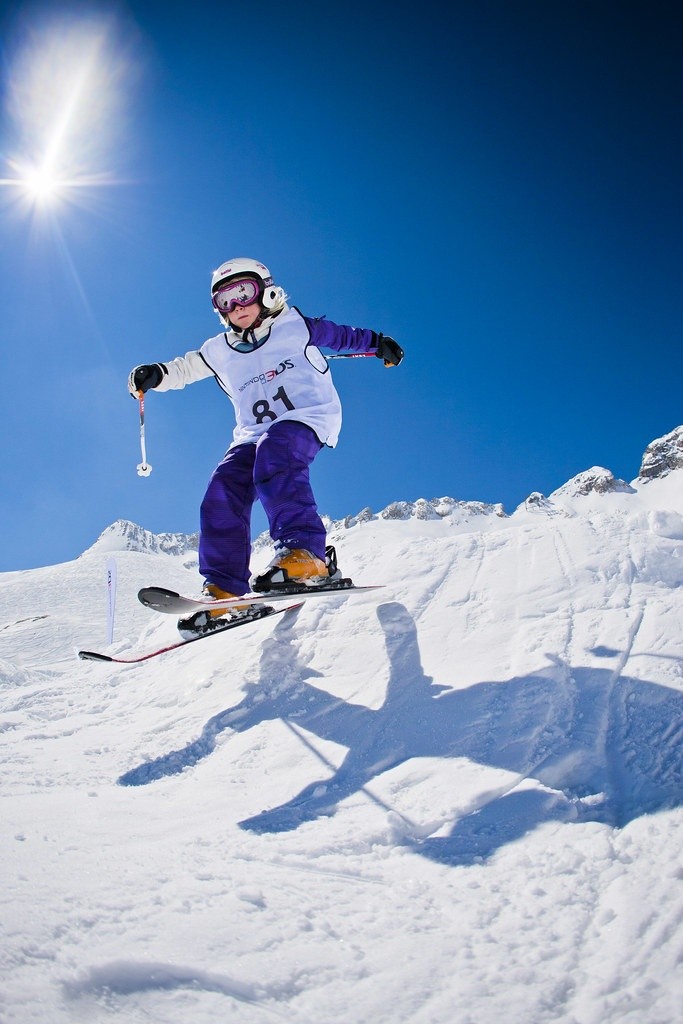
[127,258,403,638]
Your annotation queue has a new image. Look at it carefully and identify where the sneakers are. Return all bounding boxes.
[269,549,329,578]
[194,585,249,617]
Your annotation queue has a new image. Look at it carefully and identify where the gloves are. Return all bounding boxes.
[376,335,404,367]
[128,363,164,400]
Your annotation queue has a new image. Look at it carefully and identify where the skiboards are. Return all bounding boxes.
[78,599,306,665]
[137,585,388,614]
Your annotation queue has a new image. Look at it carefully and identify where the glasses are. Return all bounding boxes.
[212,280,260,312]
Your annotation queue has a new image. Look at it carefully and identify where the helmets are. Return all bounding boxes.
[210,258,285,329]
